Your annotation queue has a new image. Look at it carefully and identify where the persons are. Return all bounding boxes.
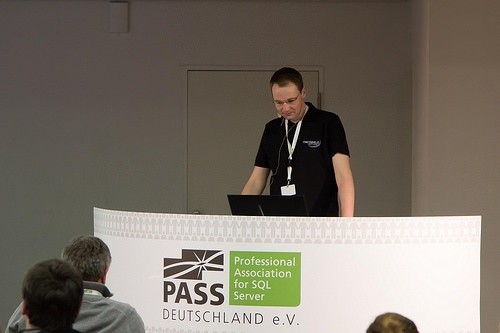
[4,235,146,333]
[240,67,355,217]
[18,259,87,333]
[367,312,419,333]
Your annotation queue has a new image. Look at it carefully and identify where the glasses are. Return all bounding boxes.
[272,93,300,106]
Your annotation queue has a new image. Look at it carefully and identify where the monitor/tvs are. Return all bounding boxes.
[227,194,309,217]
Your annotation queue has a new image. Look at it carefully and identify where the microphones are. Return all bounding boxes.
[278,113,281,118]
